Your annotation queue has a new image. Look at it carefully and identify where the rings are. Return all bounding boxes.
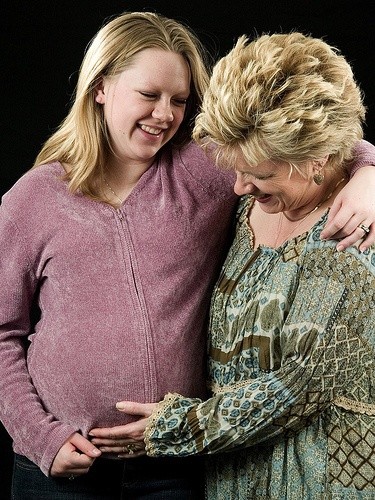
[68,473,80,481]
[123,444,137,455]
[358,223,370,233]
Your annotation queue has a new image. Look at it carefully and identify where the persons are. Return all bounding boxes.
[88,33,375,500]
[0,11,375,500]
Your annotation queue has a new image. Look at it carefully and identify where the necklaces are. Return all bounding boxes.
[103,178,122,203]
[272,175,346,249]
